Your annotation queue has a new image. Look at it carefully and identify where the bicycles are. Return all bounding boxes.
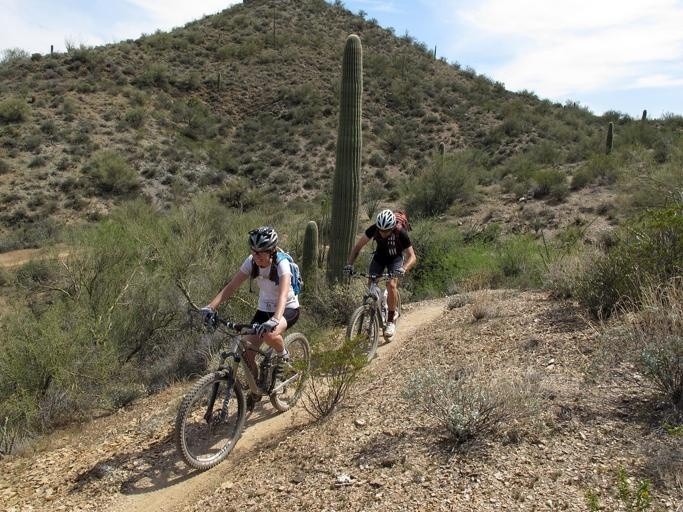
[343,270,404,368]
[177,308,312,470]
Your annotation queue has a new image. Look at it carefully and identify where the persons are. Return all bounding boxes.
[340,209,415,339]
[200,225,302,412]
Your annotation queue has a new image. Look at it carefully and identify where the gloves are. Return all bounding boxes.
[256,317,279,338]
[198,308,215,324]
[394,267,405,277]
[342,264,354,276]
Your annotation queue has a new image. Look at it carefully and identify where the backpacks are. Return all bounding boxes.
[250,252,304,296]
[374,210,409,246]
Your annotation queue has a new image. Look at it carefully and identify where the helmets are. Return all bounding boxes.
[376,209,396,230]
[248,226,278,253]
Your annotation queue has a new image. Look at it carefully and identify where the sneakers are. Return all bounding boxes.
[272,347,290,377]
[384,321,396,337]
